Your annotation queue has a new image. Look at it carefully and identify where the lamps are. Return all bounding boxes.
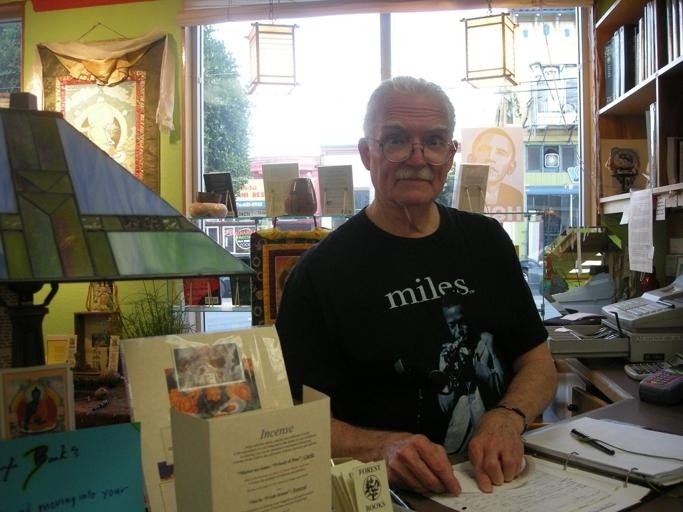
[1,87,256,367]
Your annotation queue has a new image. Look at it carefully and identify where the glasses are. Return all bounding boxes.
[368,134,459,167]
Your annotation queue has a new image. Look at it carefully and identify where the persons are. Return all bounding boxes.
[465,127,524,221]
[275,75,559,497]
[431,293,507,457]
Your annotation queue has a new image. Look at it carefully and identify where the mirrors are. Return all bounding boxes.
[540,225,639,315]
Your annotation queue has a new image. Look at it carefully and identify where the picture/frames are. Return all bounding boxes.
[249,228,333,326]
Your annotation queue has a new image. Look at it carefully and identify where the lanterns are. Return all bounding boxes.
[459,12,520,90]
[242,21,300,98]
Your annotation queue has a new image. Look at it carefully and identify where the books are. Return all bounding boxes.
[602,0,682,187]
[419,415,683,511]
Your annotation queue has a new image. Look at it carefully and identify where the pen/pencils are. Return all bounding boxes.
[572,428,615,455]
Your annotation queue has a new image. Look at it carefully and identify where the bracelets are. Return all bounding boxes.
[494,405,527,434]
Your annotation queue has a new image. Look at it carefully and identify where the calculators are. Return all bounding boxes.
[624,353,683,382]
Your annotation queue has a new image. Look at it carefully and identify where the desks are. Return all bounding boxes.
[390,358,683,512]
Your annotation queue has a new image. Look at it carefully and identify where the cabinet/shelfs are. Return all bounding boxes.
[579,0,682,225]
